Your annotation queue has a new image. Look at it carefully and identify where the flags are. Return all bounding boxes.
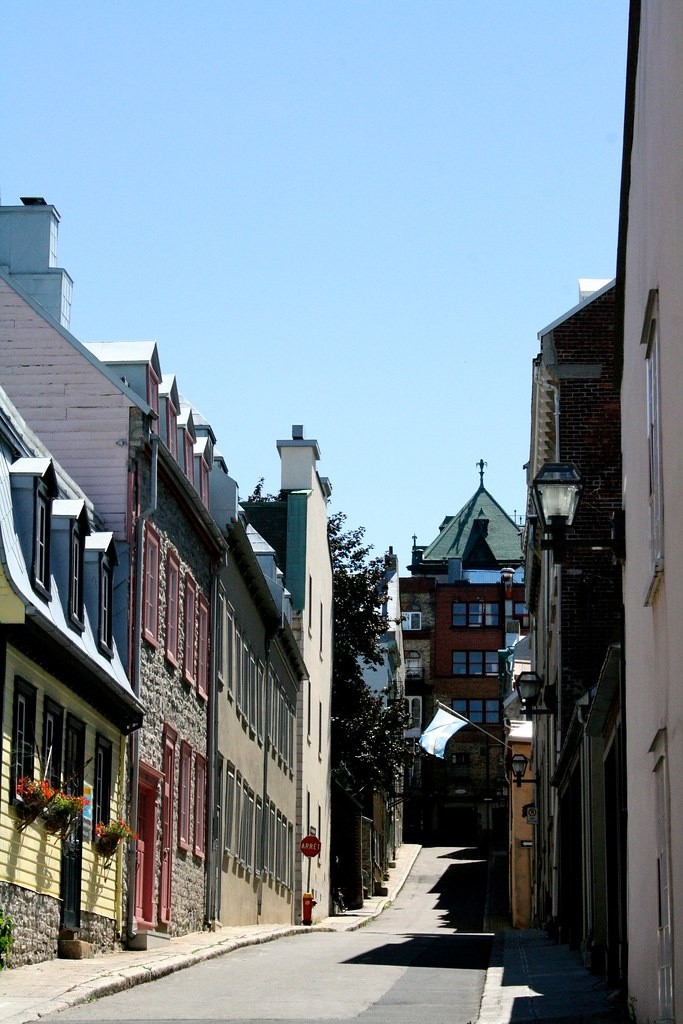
[419,708,468,759]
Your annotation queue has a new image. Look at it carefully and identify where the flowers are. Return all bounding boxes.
[54,792,90,812]
[15,777,59,804]
[96,821,139,842]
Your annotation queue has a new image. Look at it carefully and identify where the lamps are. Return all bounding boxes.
[452,755,456,763]
[510,754,539,787]
[514,671,557,721]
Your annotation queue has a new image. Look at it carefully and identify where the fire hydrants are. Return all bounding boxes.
[303,893,317,925]
